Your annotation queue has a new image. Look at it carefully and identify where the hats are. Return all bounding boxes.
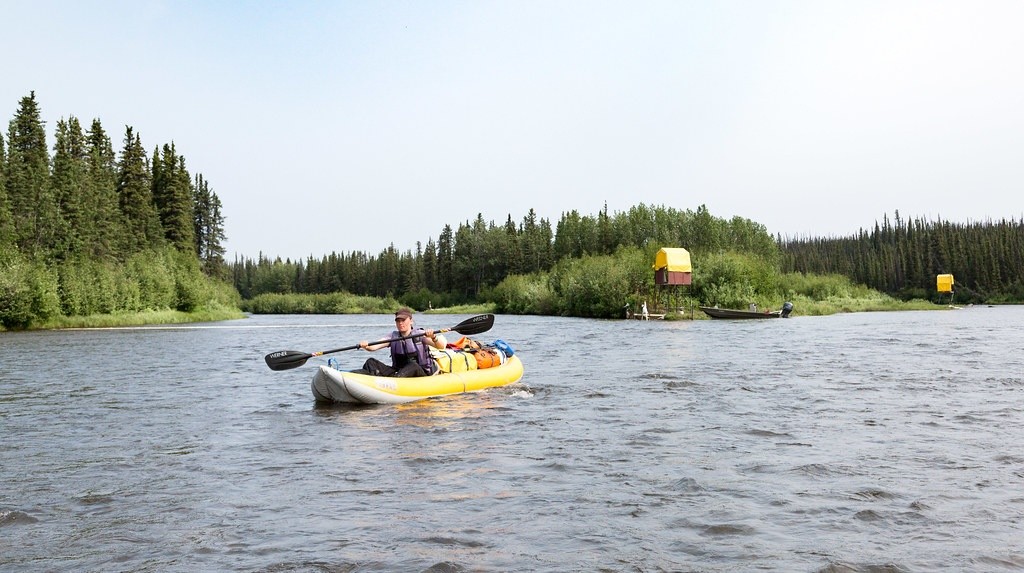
[395,307,413,321]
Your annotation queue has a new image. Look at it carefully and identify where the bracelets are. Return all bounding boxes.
[432,336,438,342]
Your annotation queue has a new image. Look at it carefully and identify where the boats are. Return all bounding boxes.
[310,339,525,405]
[698,301,793,319]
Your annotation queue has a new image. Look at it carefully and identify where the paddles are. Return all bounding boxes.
[265,314,494,372]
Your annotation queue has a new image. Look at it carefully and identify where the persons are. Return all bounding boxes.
[363,307,444,377]
